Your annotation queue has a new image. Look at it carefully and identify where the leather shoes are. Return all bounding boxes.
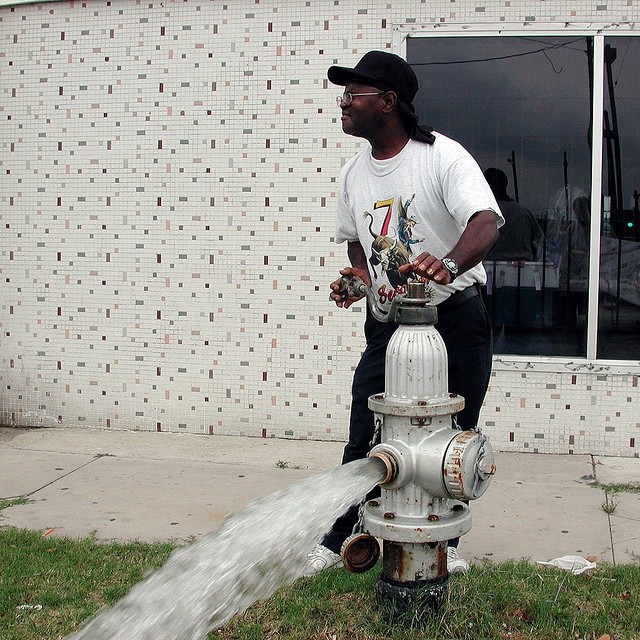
[440,283,481,306]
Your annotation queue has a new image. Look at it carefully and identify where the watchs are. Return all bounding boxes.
[443,256,460,280]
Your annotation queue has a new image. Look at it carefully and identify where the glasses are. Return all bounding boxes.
[336,91,391,108]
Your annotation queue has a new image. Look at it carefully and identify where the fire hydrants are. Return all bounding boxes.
[340,273,496,628]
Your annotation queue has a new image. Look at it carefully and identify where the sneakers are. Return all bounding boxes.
[446,547,469,576]
[302,544,345,577]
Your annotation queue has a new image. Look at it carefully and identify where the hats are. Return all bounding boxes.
[327,50,418,106]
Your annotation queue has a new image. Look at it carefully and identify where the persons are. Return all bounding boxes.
[564,197,613,298]
[475,166,544,336]
[536,158,594,287]
[324,50,504,558]
